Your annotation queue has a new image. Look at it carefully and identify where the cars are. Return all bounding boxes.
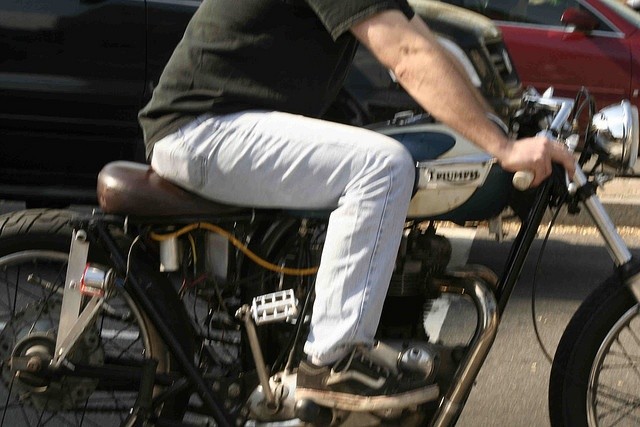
[447,1,639,163]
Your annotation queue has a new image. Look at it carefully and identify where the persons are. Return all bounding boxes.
[136,0,576,414]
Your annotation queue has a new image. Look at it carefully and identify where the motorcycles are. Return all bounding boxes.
[0,28,640,427]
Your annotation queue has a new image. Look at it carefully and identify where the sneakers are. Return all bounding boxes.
[297,344,439,412]
